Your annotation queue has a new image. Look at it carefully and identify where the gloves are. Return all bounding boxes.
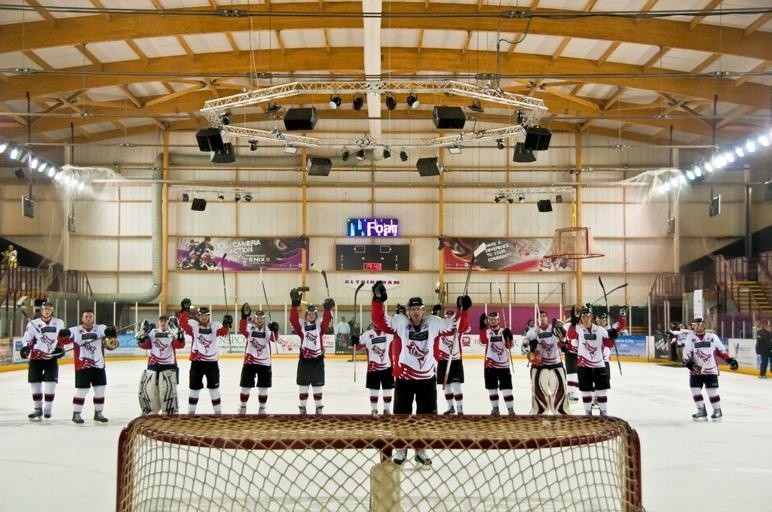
[372,281,387,301]
[223,303,252,327]
[479,305,625,349]
[51,329,71,359]
[727,357,738,370]
[267,322,280,331]
[104,325,118,338]
[457,295,472,312]
[290,288,335,309]
[139,298,192,335]
[686,361,698,371]
[20,347,30,359]
[351,334,362,346]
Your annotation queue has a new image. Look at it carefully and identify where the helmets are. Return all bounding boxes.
[41,302,56,310]
[692,318,705,325]
[407,297,424,309]
[254,310,267,318]
[197,307,212,315]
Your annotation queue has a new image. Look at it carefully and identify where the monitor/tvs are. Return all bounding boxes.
[66,213,77,234]
[707,194,721,218]
[21,194,35,220]
[664,216,677,235]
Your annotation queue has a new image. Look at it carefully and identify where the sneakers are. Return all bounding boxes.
[393,448,433,465]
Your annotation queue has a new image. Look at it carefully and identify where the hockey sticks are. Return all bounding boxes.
[221,253,232,354]
[17,296,40,331]
[498,289,517,373]
[599,276,610,326]
[354,281,366,381]
[259,267,278,356]
[311,263,329,298]
[562,283,630,322]
[37,323,136,361]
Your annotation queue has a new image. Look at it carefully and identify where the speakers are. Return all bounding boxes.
[431,105,466,129]
[537,199,552,213]
[305,158,332,176]
[34,298,49,318]
[210,142,235,163]
[208,128,225,154]
[520,124,552,151]
[195,129,211,152]
[513,142,536,162]
[190,198,206,211]
[283,107,318,130]
[415,156,441,177]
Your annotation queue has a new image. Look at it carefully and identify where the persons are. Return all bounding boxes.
[569,304,616,415]
[239,302,281,414]
[178,297,233,414]
[1,244,20,270]
[479,311,515,414]
[180,237,217,271]
[432,303,467,415]
[289,287,335,415]
[558,314,581,403]
[664,321,692,361]
[752,320,761,339]
[352,322,394,416]
[370,281,472,467]
[754,324,771,379]
[337,316,351,346]
[593,308,629,378]
[347,317,358,347]
[682,318,737,419]
[20,300,67,419]
[136,315,184,415]
[520,310,568,415]
[524,319,534,332]
[58,310,119,424]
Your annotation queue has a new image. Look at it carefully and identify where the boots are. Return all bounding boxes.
[692,408,723,418]
[443,407,517,415]
[28,408,108,423]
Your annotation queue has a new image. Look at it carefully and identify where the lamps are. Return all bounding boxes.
[218,111,263,157]
[178,189,257,205]
[463,95,527,151]
[0,138,93,195]
[327,92,423,113]
[493,189,529,204]
[658,127,772,194]
[336,146,410,162]
